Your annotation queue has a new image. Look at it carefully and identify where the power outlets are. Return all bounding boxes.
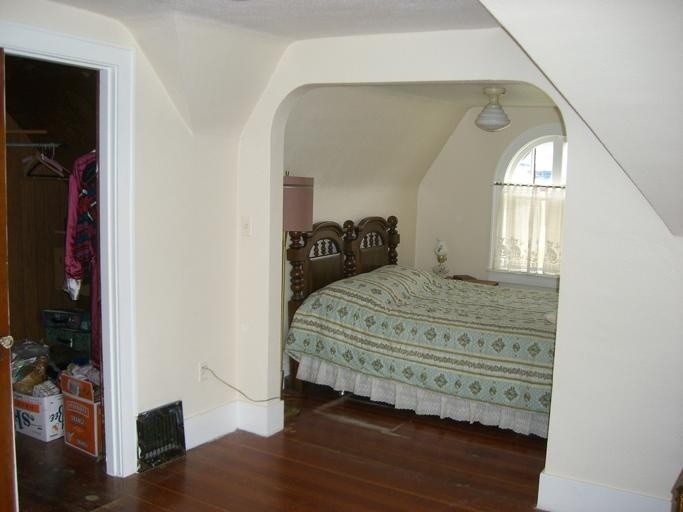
[198,359,208,381]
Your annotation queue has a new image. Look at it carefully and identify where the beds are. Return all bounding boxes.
[288,216,558,439]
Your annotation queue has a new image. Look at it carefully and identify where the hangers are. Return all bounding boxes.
[24,142,96,180]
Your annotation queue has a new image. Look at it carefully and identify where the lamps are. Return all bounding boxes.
[431,239,450,278]
[475,87,512,132]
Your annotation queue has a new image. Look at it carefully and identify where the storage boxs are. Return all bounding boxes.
[12,391,65,442]
[60,373,103,457]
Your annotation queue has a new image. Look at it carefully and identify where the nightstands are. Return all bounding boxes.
[448,274,499,286]
